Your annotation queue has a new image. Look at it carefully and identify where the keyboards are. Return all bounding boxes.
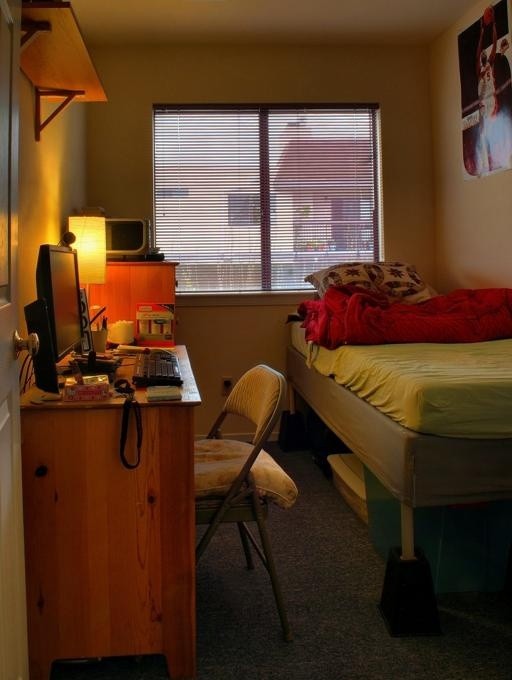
[132,352,183,386]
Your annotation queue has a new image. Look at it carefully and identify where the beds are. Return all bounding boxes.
[279,306,511,636]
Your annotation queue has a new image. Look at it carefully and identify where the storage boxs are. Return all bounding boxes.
[361,463,512,596]
[325,452,367,523]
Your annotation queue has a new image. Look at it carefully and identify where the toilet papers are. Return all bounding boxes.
[108,320,134,344]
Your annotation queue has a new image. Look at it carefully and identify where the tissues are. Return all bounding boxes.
[61,373,112,402]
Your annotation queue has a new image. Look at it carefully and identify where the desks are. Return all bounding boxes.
[16,341,207,676]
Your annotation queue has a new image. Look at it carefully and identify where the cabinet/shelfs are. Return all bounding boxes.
[79,258,182,346]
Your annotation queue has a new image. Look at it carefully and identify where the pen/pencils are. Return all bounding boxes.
[97,315,108,331]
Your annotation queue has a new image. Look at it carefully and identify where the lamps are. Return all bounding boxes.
[66,214,109,307]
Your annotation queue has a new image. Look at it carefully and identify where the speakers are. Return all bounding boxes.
[24,298,60,395]
[75,288,93,354]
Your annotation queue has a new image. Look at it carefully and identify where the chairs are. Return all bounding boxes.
[188,360,302,647]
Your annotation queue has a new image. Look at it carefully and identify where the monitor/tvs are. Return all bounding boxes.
[36,244,81,363]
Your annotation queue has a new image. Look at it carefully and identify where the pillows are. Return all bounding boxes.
[300,256,442,308]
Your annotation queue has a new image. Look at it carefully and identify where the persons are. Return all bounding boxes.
[474,10,500,170]
